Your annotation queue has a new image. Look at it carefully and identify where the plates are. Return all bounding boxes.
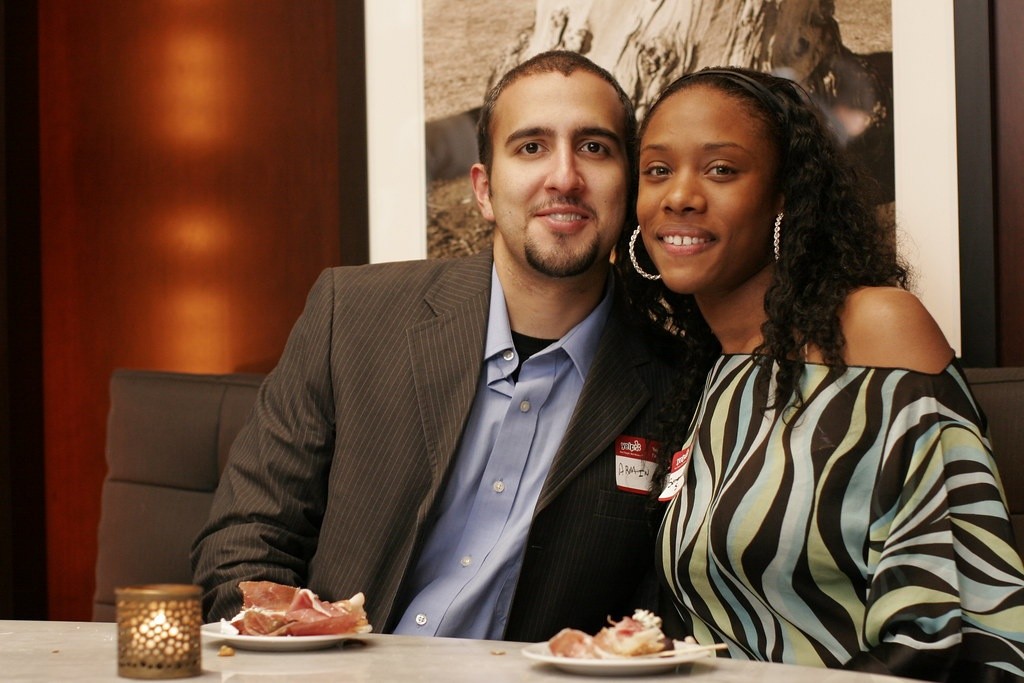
[520,640,713,675]
[199,620,370,652]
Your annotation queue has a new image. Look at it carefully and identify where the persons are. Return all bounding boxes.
[187,48,722,654]
[620,64,1024,683]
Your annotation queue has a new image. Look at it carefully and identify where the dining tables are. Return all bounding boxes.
[1,617,909,683]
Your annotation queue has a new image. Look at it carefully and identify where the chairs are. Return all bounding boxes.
[90,367,260,621]
[964,365,1024,559]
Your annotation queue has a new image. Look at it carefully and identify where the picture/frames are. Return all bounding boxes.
[335,0,998,368]
